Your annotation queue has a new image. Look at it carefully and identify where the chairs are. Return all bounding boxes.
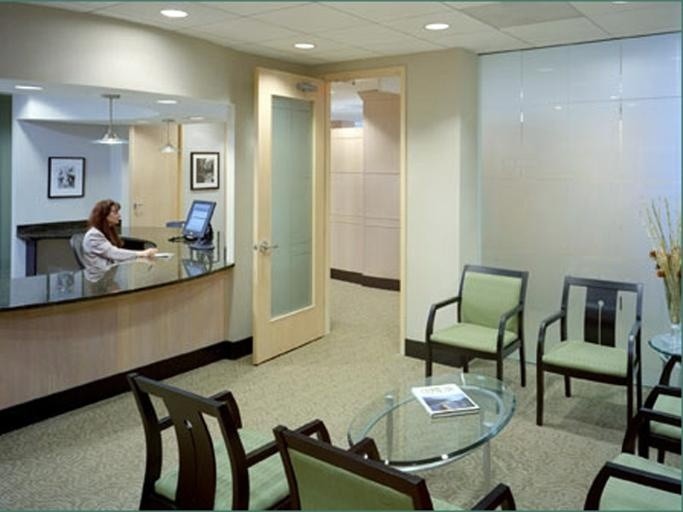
[536,274,644,427]
[272,424,516,512]
[583,406,683,512]
[127,373,334,512]
[70,233,85,269]
[639,354,683,464]
[425,264,529,387]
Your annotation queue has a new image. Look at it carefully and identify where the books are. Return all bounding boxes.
[412,383,480,418]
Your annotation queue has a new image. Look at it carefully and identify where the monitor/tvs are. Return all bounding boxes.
[182,200,216,250]
[182,250,213,278]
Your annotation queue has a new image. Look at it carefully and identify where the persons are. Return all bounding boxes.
[84,264,121,294]
[81,199,159,267]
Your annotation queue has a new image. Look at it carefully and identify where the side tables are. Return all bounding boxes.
[647,332,683,367]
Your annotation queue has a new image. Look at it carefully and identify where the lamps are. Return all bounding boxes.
[160,120,178,154]
[92,94,130,145]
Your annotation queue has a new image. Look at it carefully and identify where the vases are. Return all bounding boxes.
[669,324,683,348]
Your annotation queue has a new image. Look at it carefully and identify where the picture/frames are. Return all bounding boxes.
[47,156,85,199]
[190,151,220,190]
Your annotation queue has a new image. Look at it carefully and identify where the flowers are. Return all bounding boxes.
[642,194,683,324]
